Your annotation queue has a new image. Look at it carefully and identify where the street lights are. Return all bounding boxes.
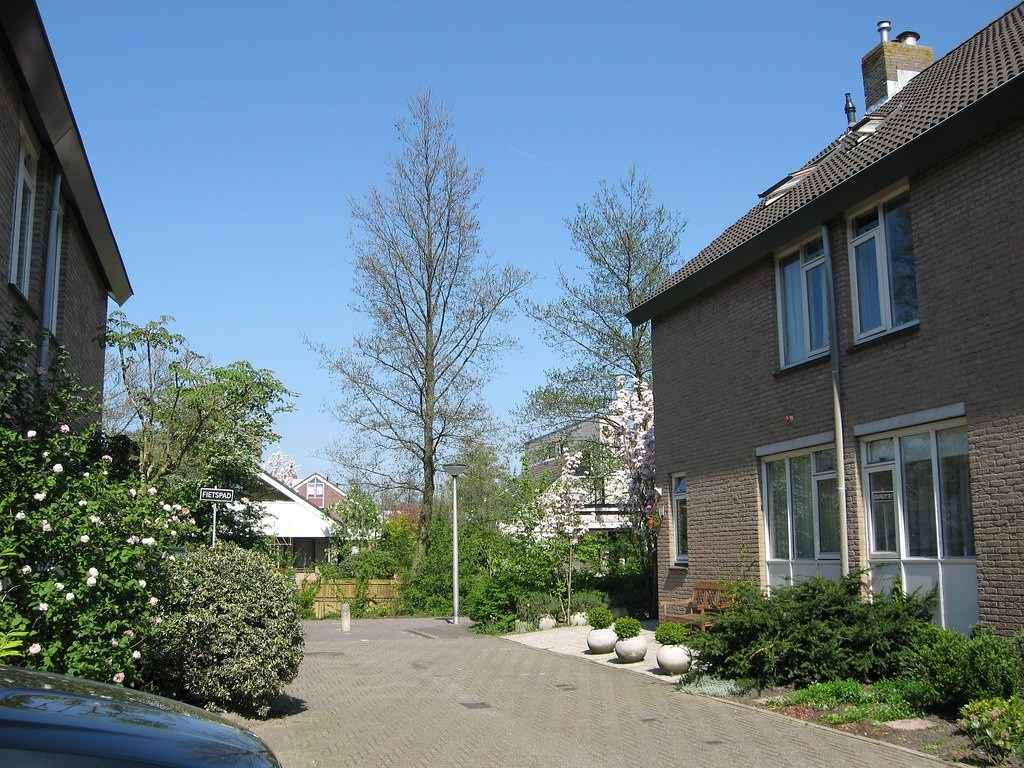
[442,463,462,624]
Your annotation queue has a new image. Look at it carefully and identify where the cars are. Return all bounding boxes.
[0,667,278,768]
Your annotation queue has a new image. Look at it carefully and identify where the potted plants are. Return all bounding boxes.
[587,603,617,653]
[612,616,648,663]
[656,620,693,672]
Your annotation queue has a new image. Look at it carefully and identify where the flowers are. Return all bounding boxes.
[646,504,662,532]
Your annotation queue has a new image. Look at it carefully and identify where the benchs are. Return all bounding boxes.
[662,582,737,632]
